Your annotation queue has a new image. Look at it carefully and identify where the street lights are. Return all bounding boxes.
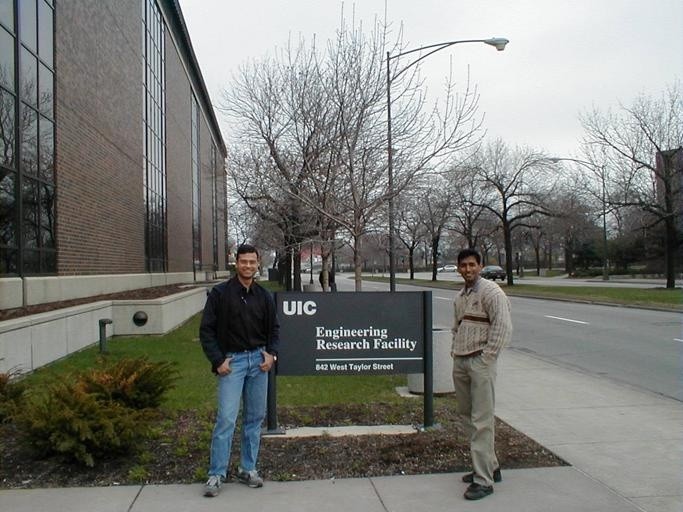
[549,157,609,280]
[385,37,509,292]
[329,146,396,292]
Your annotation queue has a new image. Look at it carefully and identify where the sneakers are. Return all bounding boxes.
[237,468,263,489]
[463,484,494,500]
[204,476,226,496]
[463,468,502,483]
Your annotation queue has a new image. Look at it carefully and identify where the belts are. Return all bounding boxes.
[220,342,267,354]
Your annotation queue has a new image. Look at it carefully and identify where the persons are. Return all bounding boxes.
[199,244,280,497]
[319,269,331,292]
[450,249,512,500]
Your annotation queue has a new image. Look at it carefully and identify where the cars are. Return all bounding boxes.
[437,264,457,273]
[478,265,506,281]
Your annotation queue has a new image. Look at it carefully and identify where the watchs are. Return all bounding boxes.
[273,355,277,363]
